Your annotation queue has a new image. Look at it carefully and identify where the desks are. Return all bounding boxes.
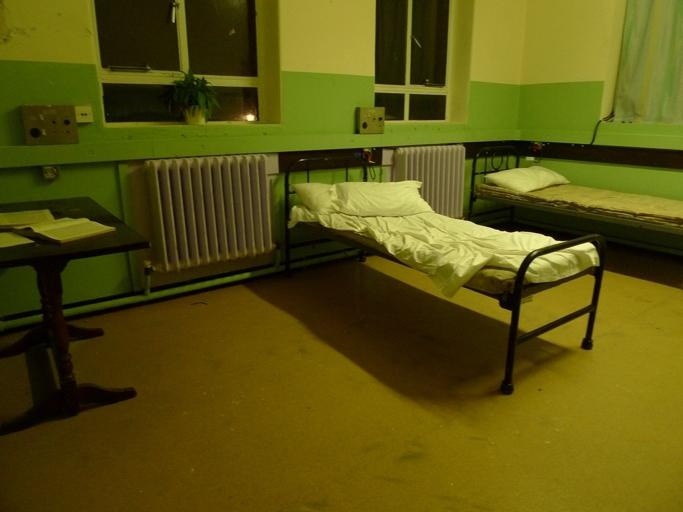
[0,196,152,435]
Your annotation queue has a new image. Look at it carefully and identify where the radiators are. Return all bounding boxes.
[140,154,275,275]
[392,145,465,220]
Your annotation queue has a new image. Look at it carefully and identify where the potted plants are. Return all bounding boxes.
[167,68,221,124]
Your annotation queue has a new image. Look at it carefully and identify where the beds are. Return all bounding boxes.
[285,155,605,397]
[467,145,683,257]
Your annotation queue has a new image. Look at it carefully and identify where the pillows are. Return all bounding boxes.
[482,165,571,193]
[288,179,435,218]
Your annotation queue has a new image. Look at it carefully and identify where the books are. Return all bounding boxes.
[0,209,56,230]
[10,217,117,244]
